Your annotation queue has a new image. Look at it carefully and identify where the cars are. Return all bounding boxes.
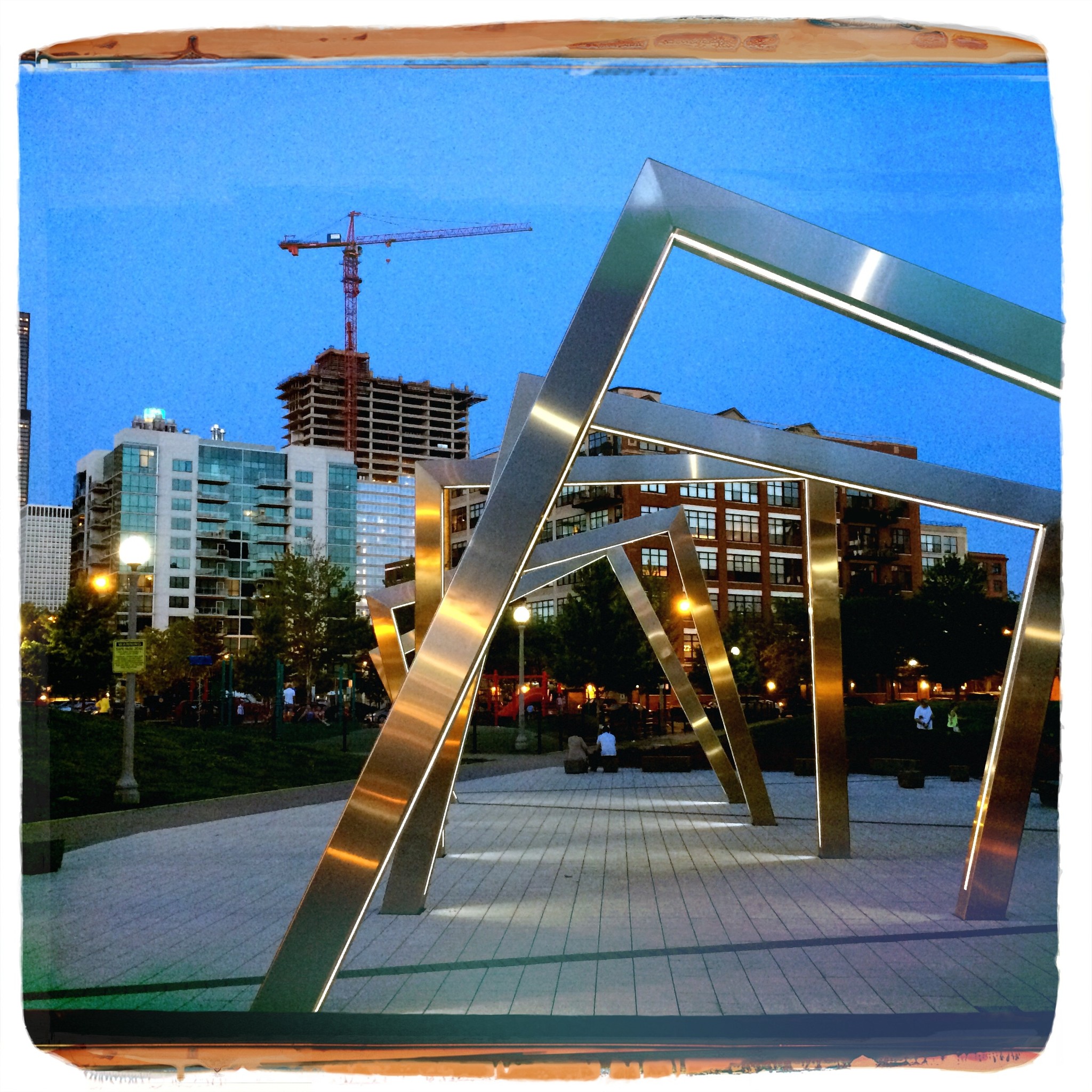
[57,700,97,713]
[364,707,390,723]
[324,701,378,722]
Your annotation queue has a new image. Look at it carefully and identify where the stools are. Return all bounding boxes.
[21,838,65,875]
[949,765,969,782]
[898,770,925,788]
[1039,780,1059,807]
[601,759,618,772]
[564,760,589,774]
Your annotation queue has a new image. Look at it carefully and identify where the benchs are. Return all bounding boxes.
[795,758,849,777]
[870,757,916,776]
[642,756,690,773]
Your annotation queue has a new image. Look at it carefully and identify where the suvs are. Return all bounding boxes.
[739,694,775,710]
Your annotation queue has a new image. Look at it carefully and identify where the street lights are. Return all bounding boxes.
[112,533,152,804]
[513,605,530,751]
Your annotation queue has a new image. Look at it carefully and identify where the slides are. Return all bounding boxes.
[497,688,543,719]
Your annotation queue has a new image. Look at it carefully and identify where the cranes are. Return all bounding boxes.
[278,210,535,455]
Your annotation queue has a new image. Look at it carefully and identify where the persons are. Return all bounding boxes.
[597,726,617,760]
[914,698,936,759]
[563,732,593,766]
[282,684,296,715]
[947,703,960,737]
[476,680,566,720]
